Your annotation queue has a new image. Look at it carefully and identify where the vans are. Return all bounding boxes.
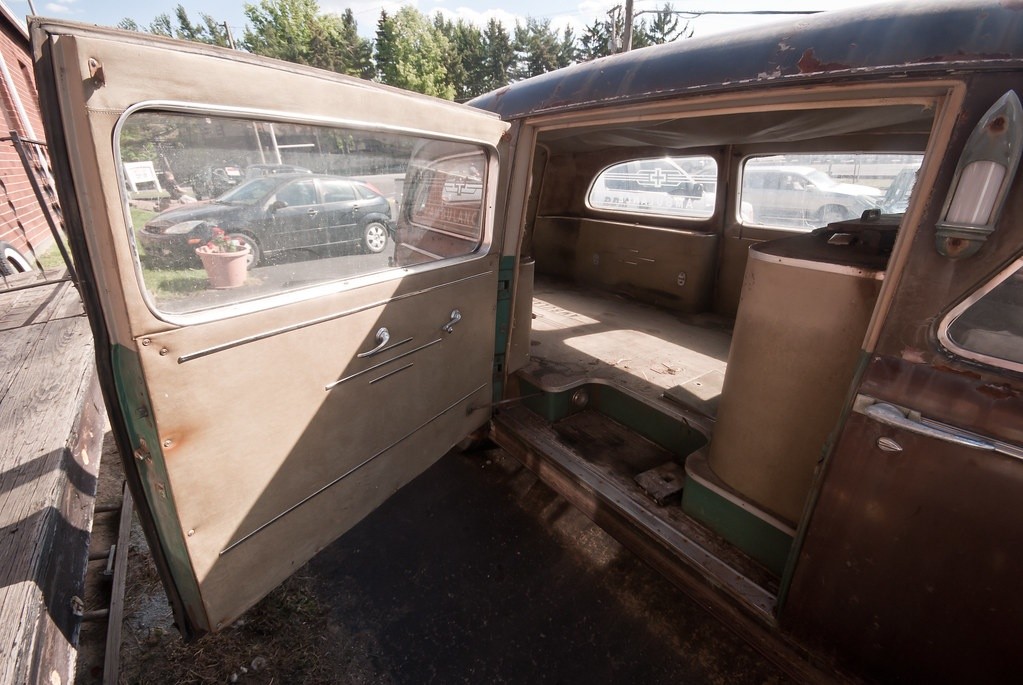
[17,0,1023,685]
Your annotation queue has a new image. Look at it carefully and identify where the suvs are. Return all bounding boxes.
[743,165,885,228]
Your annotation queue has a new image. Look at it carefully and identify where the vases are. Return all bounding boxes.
[195,246,253,286]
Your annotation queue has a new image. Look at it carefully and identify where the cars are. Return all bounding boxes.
[124,162,314,201]
[583,158,755,234]
[138,172,393,272]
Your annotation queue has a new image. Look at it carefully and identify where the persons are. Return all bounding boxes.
[165,172,197,203]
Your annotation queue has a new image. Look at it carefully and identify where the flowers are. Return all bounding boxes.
[206,228,244,252]
